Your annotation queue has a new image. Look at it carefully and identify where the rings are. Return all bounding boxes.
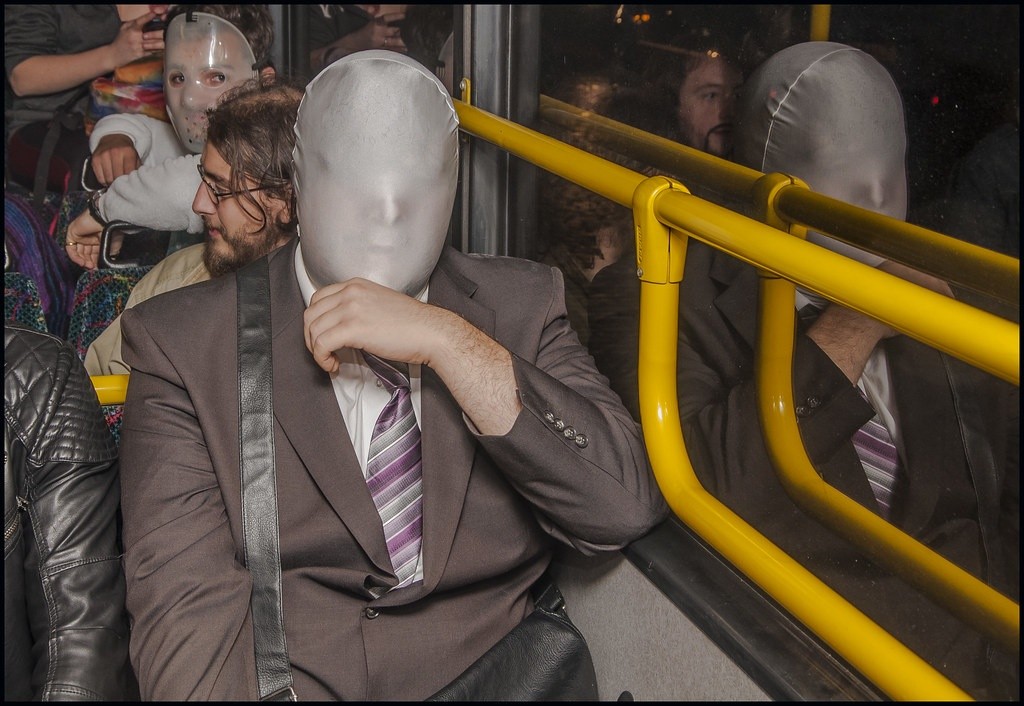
[66,243,76,245]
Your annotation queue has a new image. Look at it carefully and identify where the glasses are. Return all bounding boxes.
[196,163,288,205]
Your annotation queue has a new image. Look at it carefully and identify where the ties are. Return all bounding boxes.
[358,348,424,590]
[850,341,906,524]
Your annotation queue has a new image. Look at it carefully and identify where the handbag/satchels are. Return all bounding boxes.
[422,606,599,701]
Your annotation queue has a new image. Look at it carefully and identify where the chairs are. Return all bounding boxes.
[0,77,157,442]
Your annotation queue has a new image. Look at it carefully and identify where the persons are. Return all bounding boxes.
[4,318,119,702]
[4,4,182,191]
[308,5,1022,704]
[85,82,305,377]
[119,49,671,701]
[66,5,275,269]
